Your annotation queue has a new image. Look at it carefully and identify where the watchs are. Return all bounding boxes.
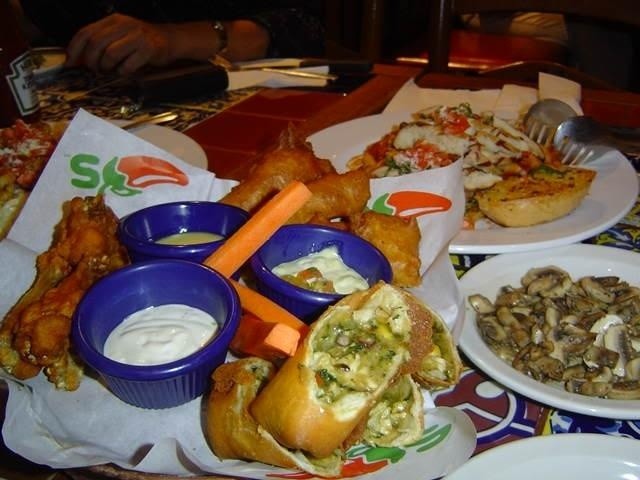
[202,14,229,58]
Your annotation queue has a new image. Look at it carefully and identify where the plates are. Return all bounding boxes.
[440,432,639,480]
[458,244,640,421]
[305,115,639,256]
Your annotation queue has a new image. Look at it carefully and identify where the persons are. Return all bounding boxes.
[16,0,327,74]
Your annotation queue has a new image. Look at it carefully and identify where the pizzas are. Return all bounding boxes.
[347,103,597,231]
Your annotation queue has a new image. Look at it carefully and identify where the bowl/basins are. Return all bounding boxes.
[68,259,243,411]
[118,201,254,280]
[249,222,394,326]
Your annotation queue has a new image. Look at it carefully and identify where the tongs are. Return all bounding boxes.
[521,95,638,167]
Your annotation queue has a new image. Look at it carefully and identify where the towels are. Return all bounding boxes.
[385,72,581,119]
